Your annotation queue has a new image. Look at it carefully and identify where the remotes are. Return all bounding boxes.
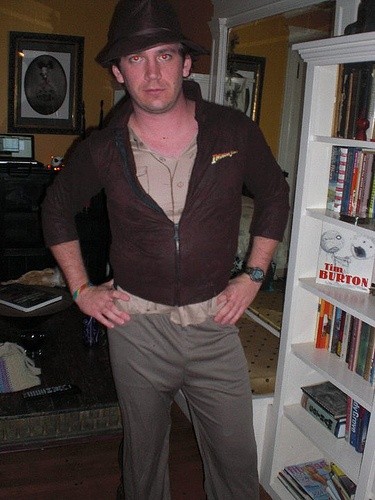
[22,383,82,401]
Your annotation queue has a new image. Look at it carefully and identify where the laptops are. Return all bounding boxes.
[0,133,38,165]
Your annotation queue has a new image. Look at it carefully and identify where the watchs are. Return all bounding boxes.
[245,267,266,282]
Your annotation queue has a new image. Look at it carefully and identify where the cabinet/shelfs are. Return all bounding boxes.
[0,167,112,279]
[258,30,375,500]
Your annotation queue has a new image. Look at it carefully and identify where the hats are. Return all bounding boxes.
[94,0,211,64]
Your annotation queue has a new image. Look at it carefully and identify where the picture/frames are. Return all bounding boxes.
[224,54,266,126]
[7,31,85,136]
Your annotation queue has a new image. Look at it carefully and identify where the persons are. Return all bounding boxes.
[42,0,289,500]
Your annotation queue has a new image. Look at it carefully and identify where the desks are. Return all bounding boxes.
[0,285,74,360]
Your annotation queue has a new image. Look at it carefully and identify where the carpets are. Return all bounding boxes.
[0,321,124,455]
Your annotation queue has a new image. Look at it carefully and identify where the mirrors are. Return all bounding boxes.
[207,0,362,340]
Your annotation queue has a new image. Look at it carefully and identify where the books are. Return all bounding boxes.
[277,60,375,499]
[0,282,62,313]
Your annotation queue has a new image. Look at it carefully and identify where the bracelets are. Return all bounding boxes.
[72,284,89,300]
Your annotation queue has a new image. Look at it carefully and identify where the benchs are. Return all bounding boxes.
[174,320,280,484]
[245,291,285,338]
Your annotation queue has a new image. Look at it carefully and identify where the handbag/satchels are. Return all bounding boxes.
[0,342,42,394]
